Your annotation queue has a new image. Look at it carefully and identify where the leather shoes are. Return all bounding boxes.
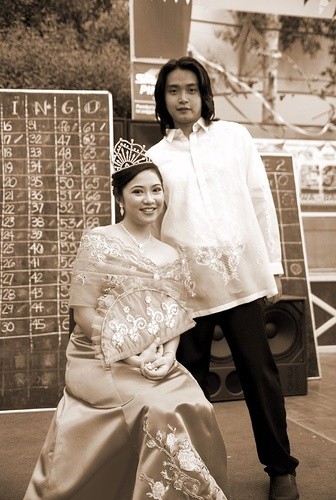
[269,475,299,499]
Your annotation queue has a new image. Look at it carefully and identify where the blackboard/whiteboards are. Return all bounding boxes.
[1,89,115,410]
[260,155,322,380]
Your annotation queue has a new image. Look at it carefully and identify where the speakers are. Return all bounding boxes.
[209,295,309,402]
